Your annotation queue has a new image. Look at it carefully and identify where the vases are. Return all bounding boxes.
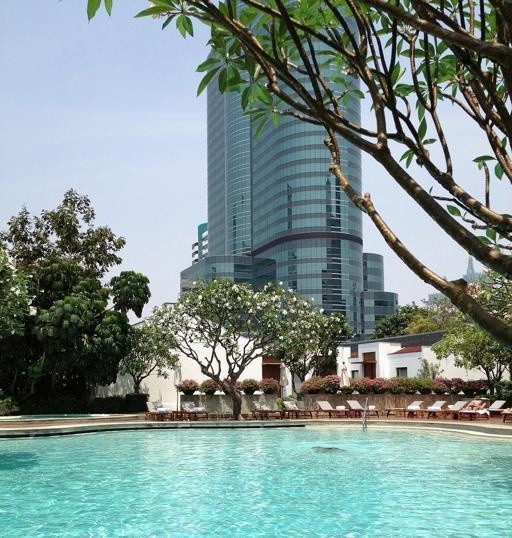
[323,386,338,394]
[449,386,460,394]
[357,386,371,394]
[392,385,402,394]
[373,386,386,394]
[224,387,237,395]
[307,387,320,394]
[404,386,418,394]
[264,388,275,394]
[463,387,475,395]
[203,388,216,395]
[420,386,432,394]
[182,388,196,395]
[243,388,255,395]
[476,387,487,395]
[434,385,446,394]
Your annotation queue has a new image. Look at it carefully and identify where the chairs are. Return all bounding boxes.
[147,400,172,421]
[469,400,505,417]
[410,401,446,419]
[250,400,280,422]
[346,400,380,419]
[182,401,208,422]
[283,399,313,418]
[385,400,424,418]
[502,409,511,422]
[316,400,348,419]
[432,401,467,421]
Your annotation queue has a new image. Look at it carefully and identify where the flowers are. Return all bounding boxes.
[357,376,489,387]
[180,379,198,388]
[221,379,236,387]
[241,378,259,387]
[260,378,278,387]
[201,379,217,387]
[302,376,342,387]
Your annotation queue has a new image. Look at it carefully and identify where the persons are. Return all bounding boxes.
[466,403,486,410]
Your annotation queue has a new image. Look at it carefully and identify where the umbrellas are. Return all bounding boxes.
[174,360,184,411]
[280,362,289,397]
[339,362,351,408]
